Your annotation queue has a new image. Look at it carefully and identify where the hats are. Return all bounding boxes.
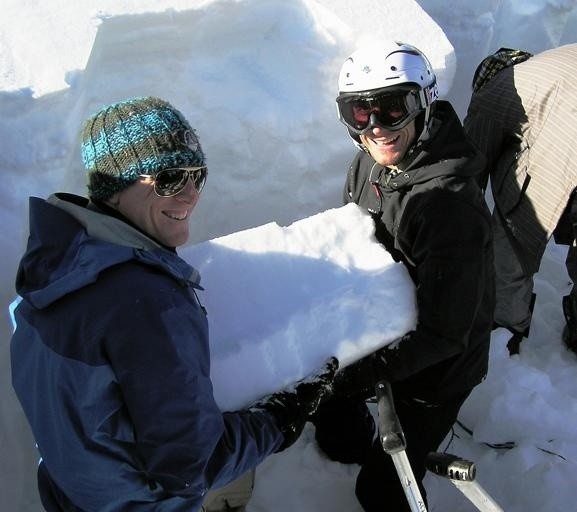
[473,48,530,90]
[79,94,204,199]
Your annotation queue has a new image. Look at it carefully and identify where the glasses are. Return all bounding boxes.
[140,166,211,199]
[335,88,419,131]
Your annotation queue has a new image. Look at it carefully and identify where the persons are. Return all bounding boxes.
[463,39,576,355]
[12,94,340,511]
[313,40,499,512]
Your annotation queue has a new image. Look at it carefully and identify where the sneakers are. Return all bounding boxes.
[493,326,524,355]
[562,320,575,355]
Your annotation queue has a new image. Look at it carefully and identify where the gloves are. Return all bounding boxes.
[249,354,344,451]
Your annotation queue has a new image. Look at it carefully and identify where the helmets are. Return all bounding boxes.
[334,38,439,149]
